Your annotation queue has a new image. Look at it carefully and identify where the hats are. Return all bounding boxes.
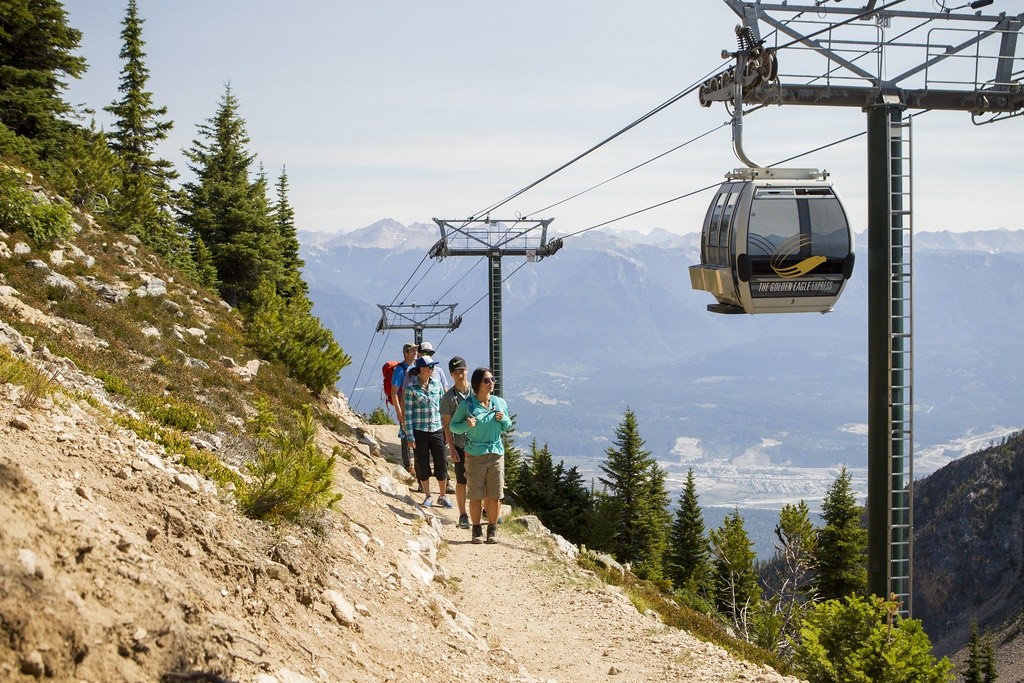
[403,343,419,353]
[417,342,435,353]
[449,356,467,372]
[415,355,440,370]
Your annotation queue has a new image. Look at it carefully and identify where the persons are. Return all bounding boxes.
[400,342,456,495]
[391,343,436,478]
[449,368,512,546]
[439,356,491,531]
[404,355,455,511]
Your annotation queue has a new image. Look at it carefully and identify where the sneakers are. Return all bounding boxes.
[459,514,469,529]
[446,484,455,494]
[487,524,497,544]
[472,525,483,544]
[421,496,433,508]
[418,484,425,492]
[436,496,453,509]
[482,509,489,520]
[407,465,416,477]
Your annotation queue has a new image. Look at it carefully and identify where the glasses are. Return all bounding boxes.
[428,365,434,368]
[481,377,495,384]
[422,351,434,355]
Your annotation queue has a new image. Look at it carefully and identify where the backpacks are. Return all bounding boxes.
[382,360,407,411]
